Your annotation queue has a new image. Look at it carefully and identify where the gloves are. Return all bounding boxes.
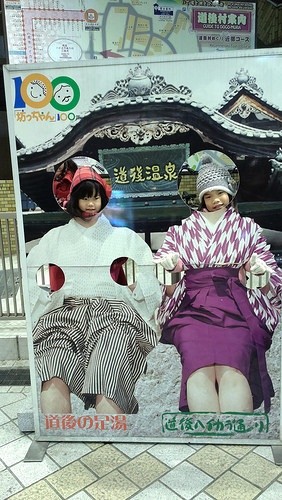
[154,252,179,269]
[250,253,267,274]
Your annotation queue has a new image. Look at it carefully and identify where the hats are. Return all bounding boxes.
[69,166,111,198]
[196,153,236,203]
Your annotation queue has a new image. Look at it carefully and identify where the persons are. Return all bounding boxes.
[161,154,268,287]
[47,158,129,290]
